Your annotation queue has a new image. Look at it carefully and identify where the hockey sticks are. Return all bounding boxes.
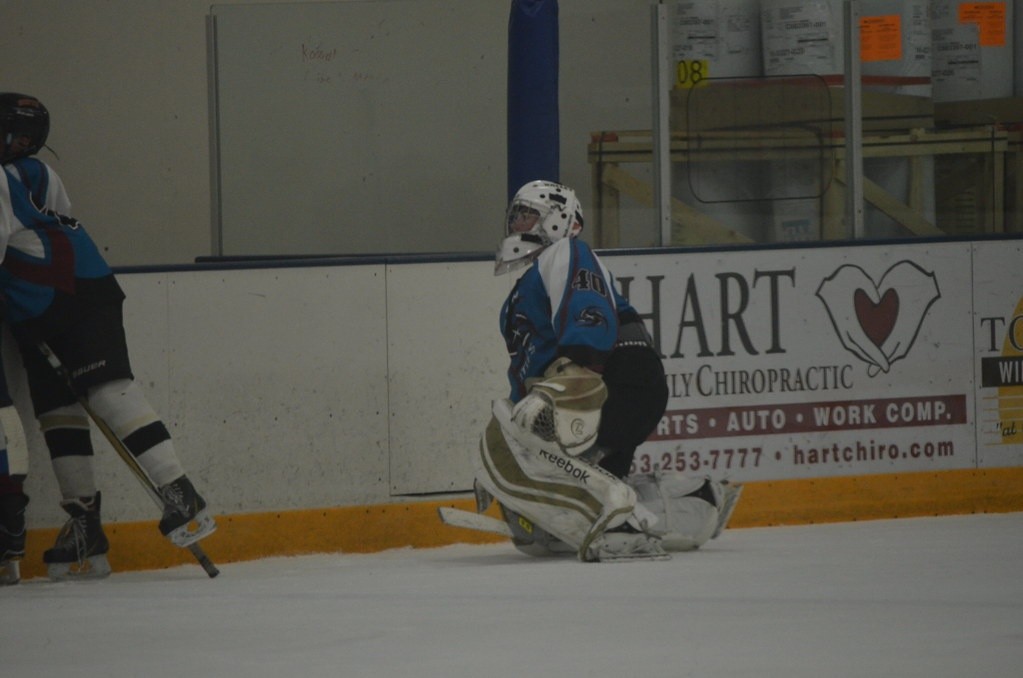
[435,505,517,542]
[36,341,222,580]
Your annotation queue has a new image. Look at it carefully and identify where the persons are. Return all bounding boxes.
[0,94,215,583]
[480,179,739,562]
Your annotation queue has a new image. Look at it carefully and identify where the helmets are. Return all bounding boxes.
[494,179,584,275]
[0,93,50,165]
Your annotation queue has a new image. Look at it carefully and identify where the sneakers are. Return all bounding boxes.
[157,476,216,547]
[42,491,110,578]
[0,494,31,584]
